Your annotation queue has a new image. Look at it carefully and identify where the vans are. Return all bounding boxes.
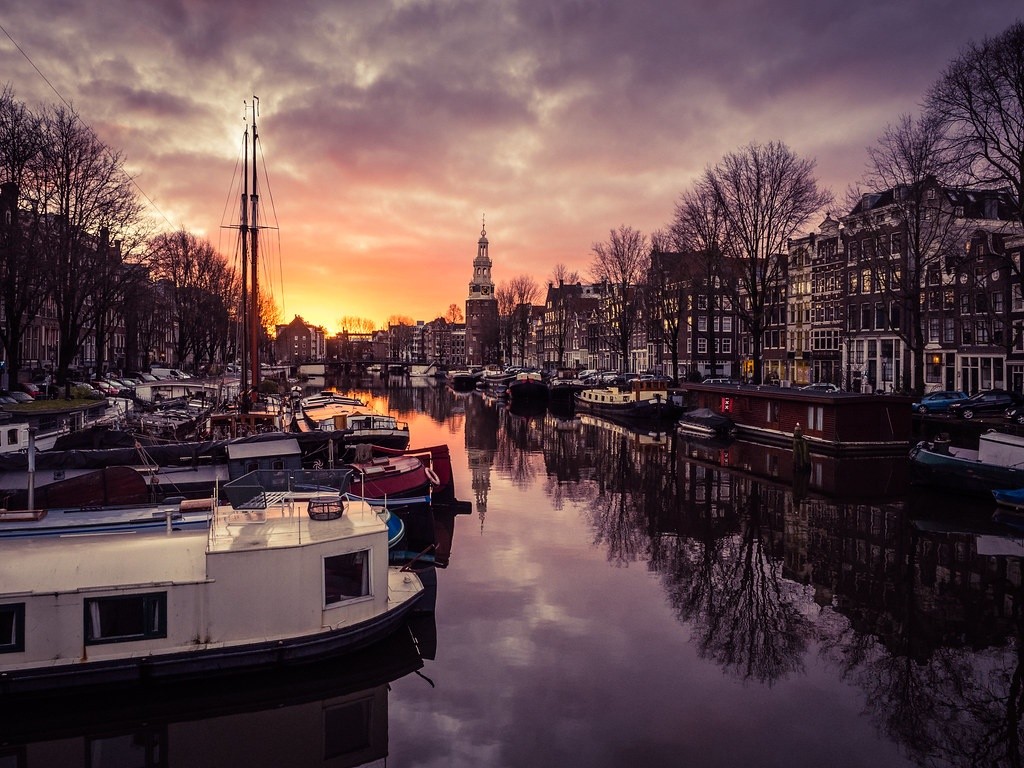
[703,378,741,386]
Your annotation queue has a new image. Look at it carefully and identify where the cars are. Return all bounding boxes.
[101,367,190,396]
[1001,400,1024,424]
[799,382,840,393]
[946,388,1024,420]
[68,380,105,401]
[628,374,656,385]
[912,390,970,415]
[594,371,619,385]
[91,380,120,397]
[573,369,598,380]
[18,381,46,400]
[0,390,36,404]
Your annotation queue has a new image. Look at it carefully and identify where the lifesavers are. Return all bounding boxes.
[424,467,441,486]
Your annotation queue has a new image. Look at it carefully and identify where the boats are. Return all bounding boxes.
[0,93,1024,699]
